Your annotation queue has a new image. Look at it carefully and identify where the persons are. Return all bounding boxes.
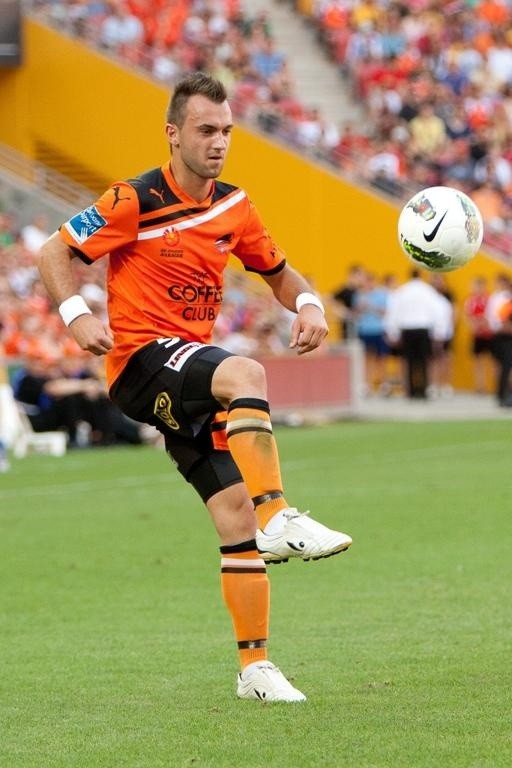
[215,274,299,358]
[0,211,164,451]
[35,0,512,255]
[32,71,356,705]
[335,265,512,409]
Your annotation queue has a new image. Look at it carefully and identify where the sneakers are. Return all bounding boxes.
[237,663,306,701]
[255,507,352,563]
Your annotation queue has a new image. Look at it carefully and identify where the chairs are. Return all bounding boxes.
[1,383,71,460]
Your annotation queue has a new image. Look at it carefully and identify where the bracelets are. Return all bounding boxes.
[295,292,323,313]
[58,294,92,326]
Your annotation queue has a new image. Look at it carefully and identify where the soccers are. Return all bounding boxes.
[398,186,484,272]
